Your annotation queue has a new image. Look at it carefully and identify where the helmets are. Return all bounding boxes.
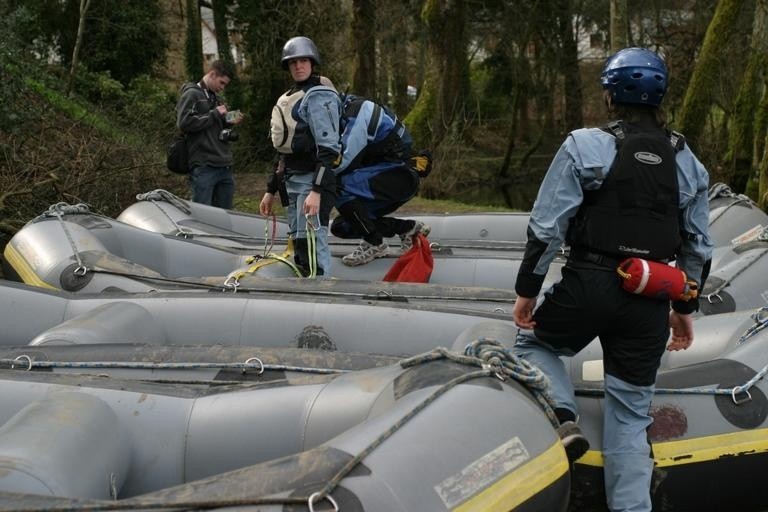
[598,46,670,107]
[281,35,320,71]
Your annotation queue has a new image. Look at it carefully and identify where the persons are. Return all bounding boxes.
[173,58,244,212]
[512,46,715,512]
[259,34,340,277]
[329,93,432,267]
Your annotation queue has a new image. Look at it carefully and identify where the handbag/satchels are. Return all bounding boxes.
[167,138,189,173]
[406,149,433,177]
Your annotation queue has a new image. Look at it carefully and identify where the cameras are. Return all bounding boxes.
[219,128,239,142]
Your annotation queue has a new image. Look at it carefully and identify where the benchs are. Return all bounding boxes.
[226,258,301,280]
[0,391,129,499]
[29,302,154,344]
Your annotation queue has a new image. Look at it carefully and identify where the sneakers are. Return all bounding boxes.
[398,223,430,256]
[556,422,589,459]
[342,240,389,267]
